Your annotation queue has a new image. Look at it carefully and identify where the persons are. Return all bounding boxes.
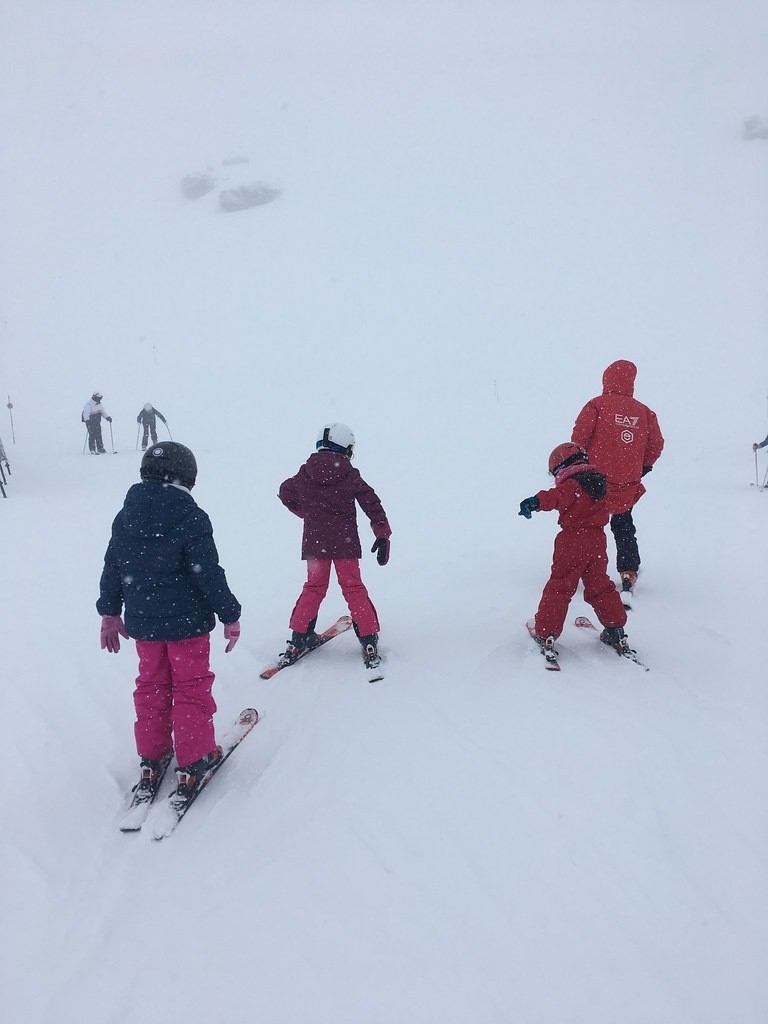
[518,441,631,649]
[753,429,768,488]
[95,440,241,802]
[277,424,393,662]
[137,403,168,452]
[572,359,665,588]
[81,391,112,455]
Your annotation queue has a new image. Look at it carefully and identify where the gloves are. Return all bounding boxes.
[106,417,112,423]
[86,420,90,426]
[372,537,390,567]
[101,615,129,653]
[163,418,165,422]
[640,466,653,478]
[137,417,142,424]
[519,496,540,518]
[224,621,240,653]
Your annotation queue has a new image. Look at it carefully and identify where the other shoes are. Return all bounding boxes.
[141,447,147,452]
[532,633,557,650]
[599,626,624,646]
[621,572,636,596]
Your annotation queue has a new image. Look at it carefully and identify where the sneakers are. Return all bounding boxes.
[98,448,106,453]
[286,631,321,657]
[139,748,174,785]
[91,450,100,455]
[360,635,382,669]
[174,745,223,794]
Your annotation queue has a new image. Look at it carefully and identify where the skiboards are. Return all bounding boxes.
[259,616,384,682]
[119,708,258,845]
[526,616,655,673]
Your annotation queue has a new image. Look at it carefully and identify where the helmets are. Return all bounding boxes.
[549,442,590,477]
[92,392,103,403]
[315,422,356,461]
[145,403,153,412]
[138,442,197,489]
